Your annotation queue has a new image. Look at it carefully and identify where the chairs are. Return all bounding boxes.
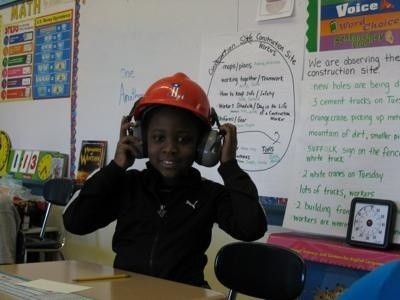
[214,241,307,299]
[16,177,73,263]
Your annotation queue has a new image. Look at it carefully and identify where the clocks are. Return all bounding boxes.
[345,196,396,250]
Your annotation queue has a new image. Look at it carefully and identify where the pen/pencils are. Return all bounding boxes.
[73,274,130,282]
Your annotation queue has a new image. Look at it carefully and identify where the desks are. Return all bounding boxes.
[0,259,226,299]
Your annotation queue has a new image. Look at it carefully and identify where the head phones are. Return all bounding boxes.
[122,92,227,167]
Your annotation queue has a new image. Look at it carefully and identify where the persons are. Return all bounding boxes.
[63,72,268,290]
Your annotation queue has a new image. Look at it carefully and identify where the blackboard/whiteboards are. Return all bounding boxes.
[71,0,399,214]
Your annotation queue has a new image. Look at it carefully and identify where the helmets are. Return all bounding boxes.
[134,72,210,124]
